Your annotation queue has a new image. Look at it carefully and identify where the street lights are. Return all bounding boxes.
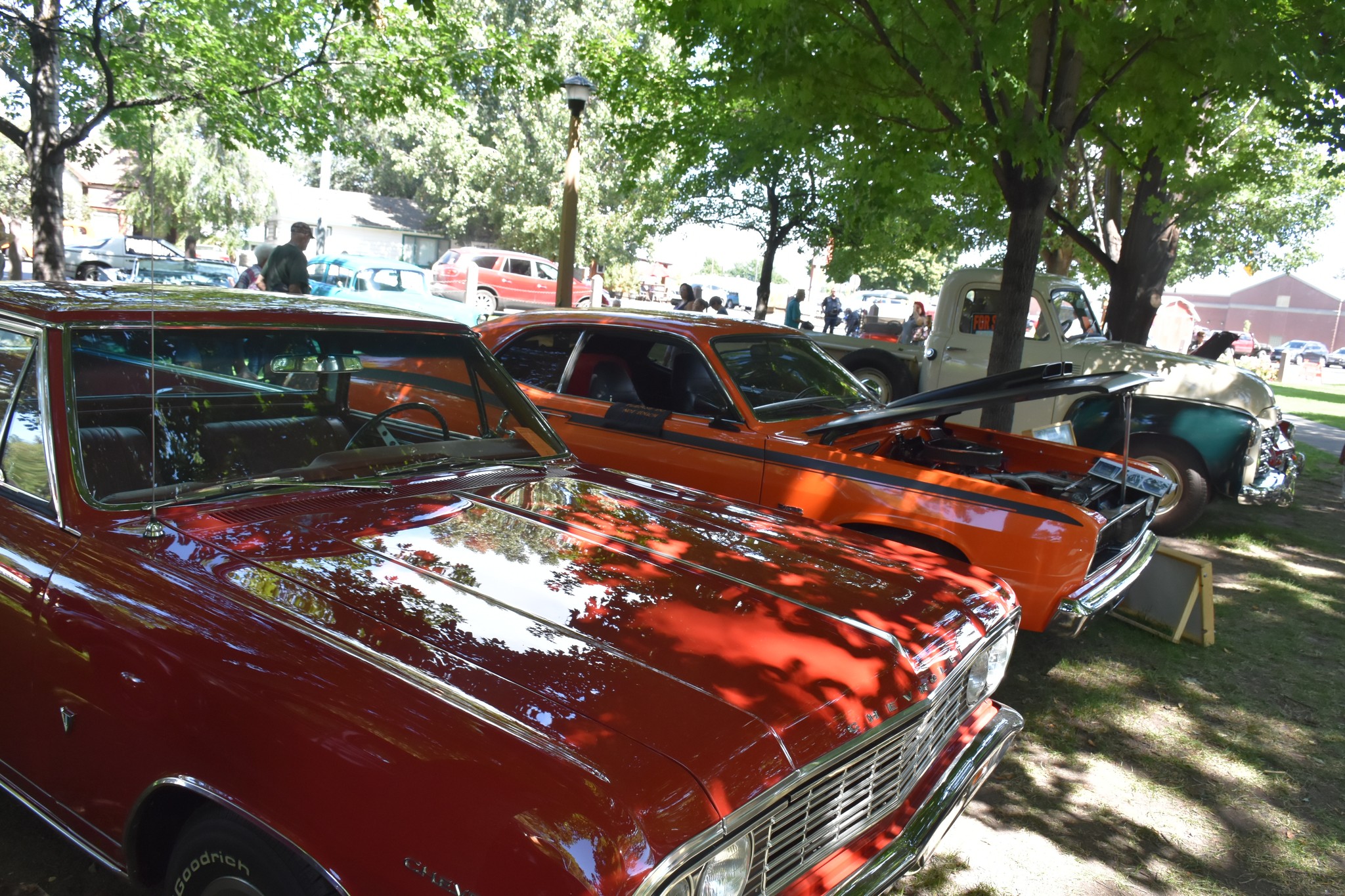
[553,72,594,308]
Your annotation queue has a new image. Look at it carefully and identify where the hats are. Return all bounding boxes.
[708,296,722,307]
[290,222,315,240]
[831,288,836,292]
[793,289,806,296]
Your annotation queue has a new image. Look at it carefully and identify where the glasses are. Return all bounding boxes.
[1202,336,1206,337]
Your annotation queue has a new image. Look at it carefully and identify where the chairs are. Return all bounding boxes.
[673,353,721,416]
[587,361,642,406]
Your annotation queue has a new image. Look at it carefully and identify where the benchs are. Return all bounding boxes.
[78,390,359,503]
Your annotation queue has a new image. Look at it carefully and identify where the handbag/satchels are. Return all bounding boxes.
[911,320,929,341]
[271,283,309,293]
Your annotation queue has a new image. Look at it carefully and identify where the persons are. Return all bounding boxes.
[692,298,706,313]
[233,243,274,291]
[673,283,695,311]
[255,222,315,296]
[901,301,926,347]
[843,308,860,339]
[821,287,842,334]
[785,289,809,330]
[1187,331,1206,355]
[709,296,728,315]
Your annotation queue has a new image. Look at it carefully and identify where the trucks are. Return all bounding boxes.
[799,268,1306,537]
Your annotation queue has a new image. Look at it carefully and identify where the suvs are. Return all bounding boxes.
[429,246,612,311]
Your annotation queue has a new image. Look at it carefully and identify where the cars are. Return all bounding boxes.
[1204,330,1345,369]
[0,279,1027,896]
[346,306,1174,644]
[304,253,487,329]
[63,233,240,289]
